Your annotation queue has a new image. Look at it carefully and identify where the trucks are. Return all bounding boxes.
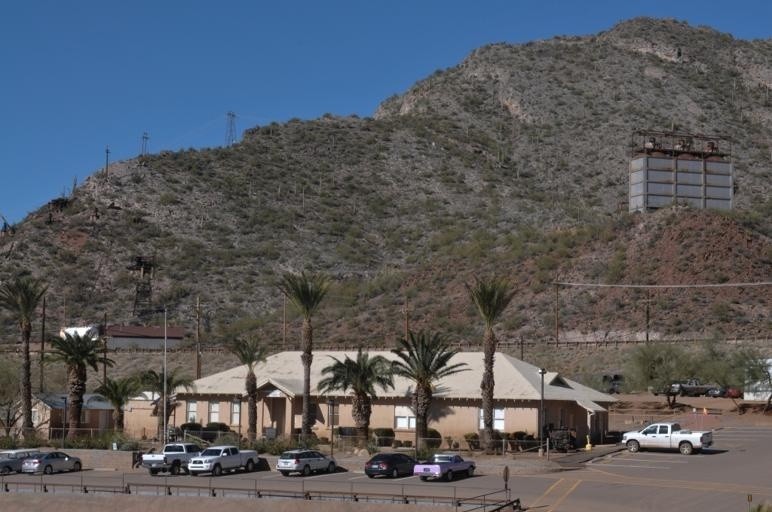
[742,358,772,404]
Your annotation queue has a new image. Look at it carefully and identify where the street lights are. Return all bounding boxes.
[59,396,68,450]
[537,366,547,456]
[325,396,335,461]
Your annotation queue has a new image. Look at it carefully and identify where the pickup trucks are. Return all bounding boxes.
[621,421,712,456]
[413,453,476,482]
[187,444,258,477]
[1,447,40,474]
[142,441,203,476]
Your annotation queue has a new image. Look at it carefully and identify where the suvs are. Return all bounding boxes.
[276,448,336,477]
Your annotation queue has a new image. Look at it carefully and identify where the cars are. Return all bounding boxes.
[364,453,417,479]
[21,451,82,475]
[156,424,183,443]
[606,378,743,399]
